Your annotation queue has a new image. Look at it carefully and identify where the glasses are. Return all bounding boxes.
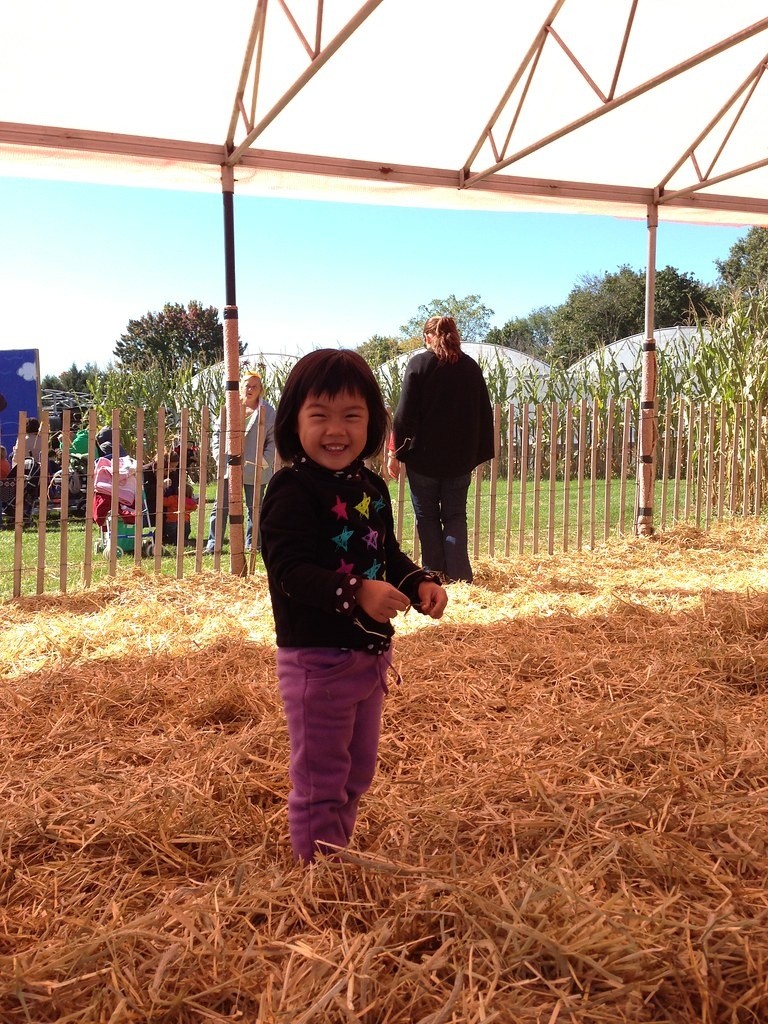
[423,342,427,349]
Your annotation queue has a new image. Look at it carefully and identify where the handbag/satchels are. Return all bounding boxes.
[69,469,81,494]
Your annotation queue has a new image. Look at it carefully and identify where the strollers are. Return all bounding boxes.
[49,459,92,518]
[0,450,48,531]
[140,440,200,484]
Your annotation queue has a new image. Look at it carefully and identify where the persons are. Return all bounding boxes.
[389,314,495,584]
[0,412,128,526]
[201,371,277,557]
[258,347,448,871]
[142,440,197,548]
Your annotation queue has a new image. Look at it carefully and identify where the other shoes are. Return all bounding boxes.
[247,548,261,555]
[202,548,214,557]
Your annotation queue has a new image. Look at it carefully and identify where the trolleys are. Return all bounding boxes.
[96,465,167,561]
